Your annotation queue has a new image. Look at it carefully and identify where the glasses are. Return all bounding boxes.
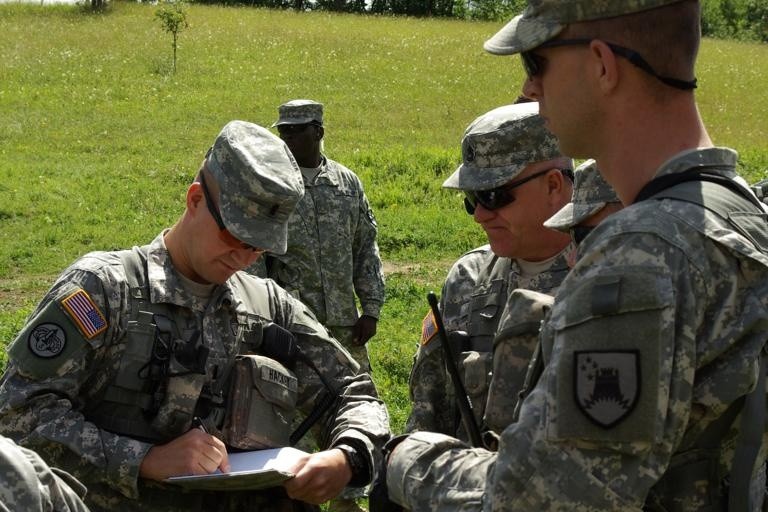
[276,123,319,134]
[566,224,597,249]
[462,166,574,216]
[517,36,699,91]
[197,168,267,255]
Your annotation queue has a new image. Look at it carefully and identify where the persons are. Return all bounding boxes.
[366,1,768,511]
[543,154,625,271]
[406,102,575,452]
[1,120,389,512]
[241,98,386,510]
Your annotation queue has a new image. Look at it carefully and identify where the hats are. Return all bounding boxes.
[540,157,624,235]
[204,118,307,258]
[480,0,705,57]
[269,98,324,129]
[439,99,564,192]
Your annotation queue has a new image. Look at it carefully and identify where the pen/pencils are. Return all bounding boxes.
[193,416,225,474]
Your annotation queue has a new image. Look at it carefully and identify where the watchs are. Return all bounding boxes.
[335,440,369,487]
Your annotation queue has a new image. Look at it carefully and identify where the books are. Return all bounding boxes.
[162,446,312,492]
